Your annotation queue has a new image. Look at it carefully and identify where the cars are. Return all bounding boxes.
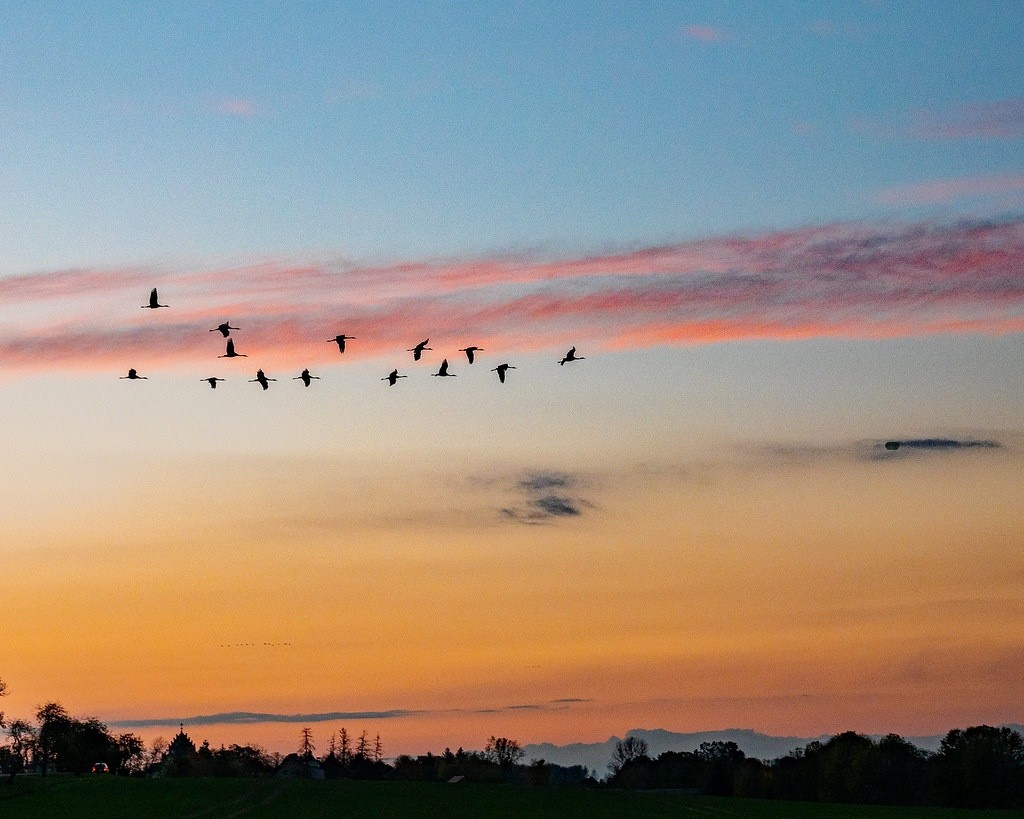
[91,763,110,774]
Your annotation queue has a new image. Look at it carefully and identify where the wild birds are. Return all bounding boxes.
[119,288,585,392]
